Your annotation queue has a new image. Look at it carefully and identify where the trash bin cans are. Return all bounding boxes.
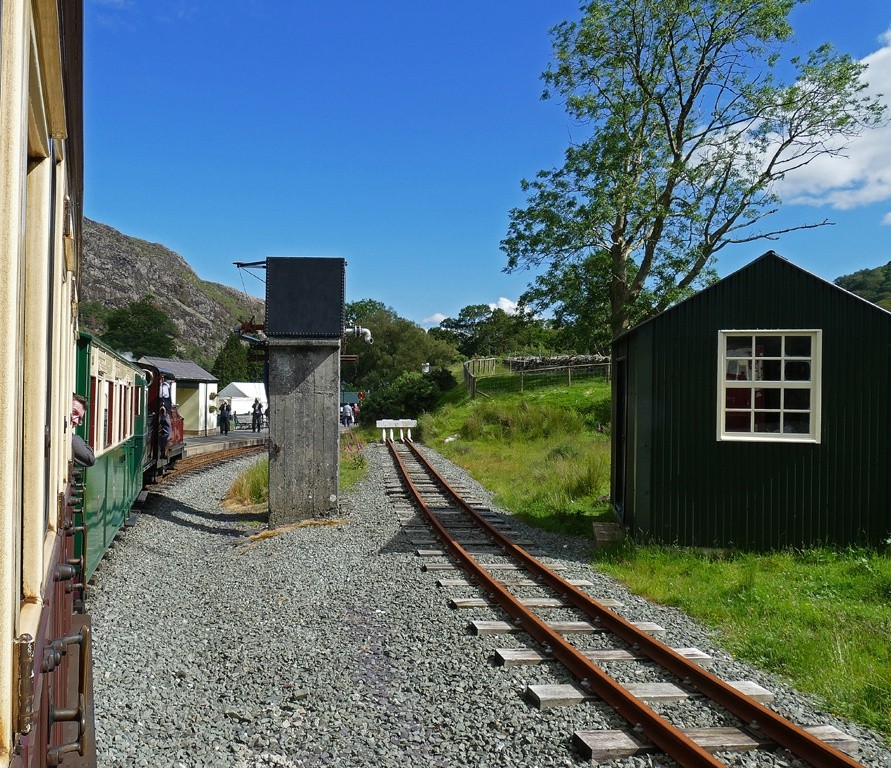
[218,415,234,431]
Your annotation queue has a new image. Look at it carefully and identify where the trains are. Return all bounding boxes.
[0,0,186,768]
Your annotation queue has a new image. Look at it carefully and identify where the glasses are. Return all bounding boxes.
[72,409,84,426]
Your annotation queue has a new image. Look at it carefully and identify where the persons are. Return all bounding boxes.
[71,392,96,467]
[159,406,172,459]
[251,398,263,433]
[342,402,352,427]
[147,405,156,458]
[352,403,360,425]
[219,400,231,436]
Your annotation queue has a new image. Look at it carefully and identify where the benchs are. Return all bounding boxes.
[233,411,268,430]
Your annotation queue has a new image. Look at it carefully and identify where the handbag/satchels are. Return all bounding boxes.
[351,416,354,423]
